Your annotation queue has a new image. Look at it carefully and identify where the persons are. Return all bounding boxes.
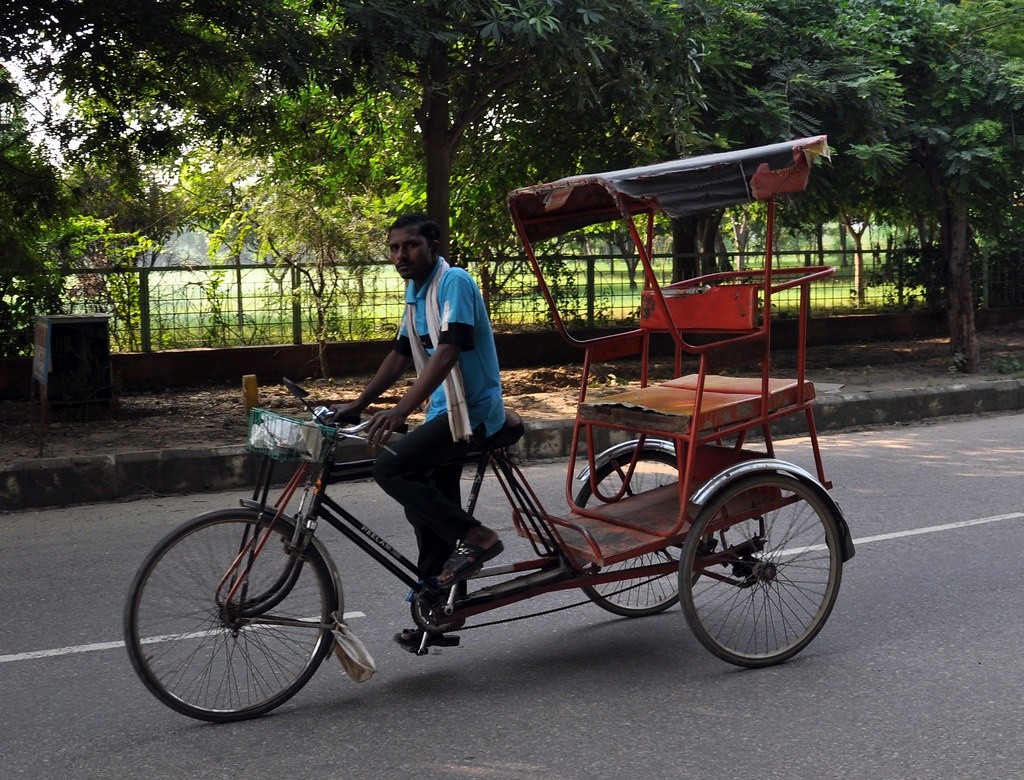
[330,214,504,648]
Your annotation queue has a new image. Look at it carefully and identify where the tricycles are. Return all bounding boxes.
[123,135,857,724]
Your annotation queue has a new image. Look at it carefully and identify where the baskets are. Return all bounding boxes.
[246,407,337,464]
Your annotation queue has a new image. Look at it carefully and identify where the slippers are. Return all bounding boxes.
[393,625,461,647]
[437,540,504,588]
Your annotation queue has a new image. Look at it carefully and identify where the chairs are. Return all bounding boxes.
[579,266,837,436]
